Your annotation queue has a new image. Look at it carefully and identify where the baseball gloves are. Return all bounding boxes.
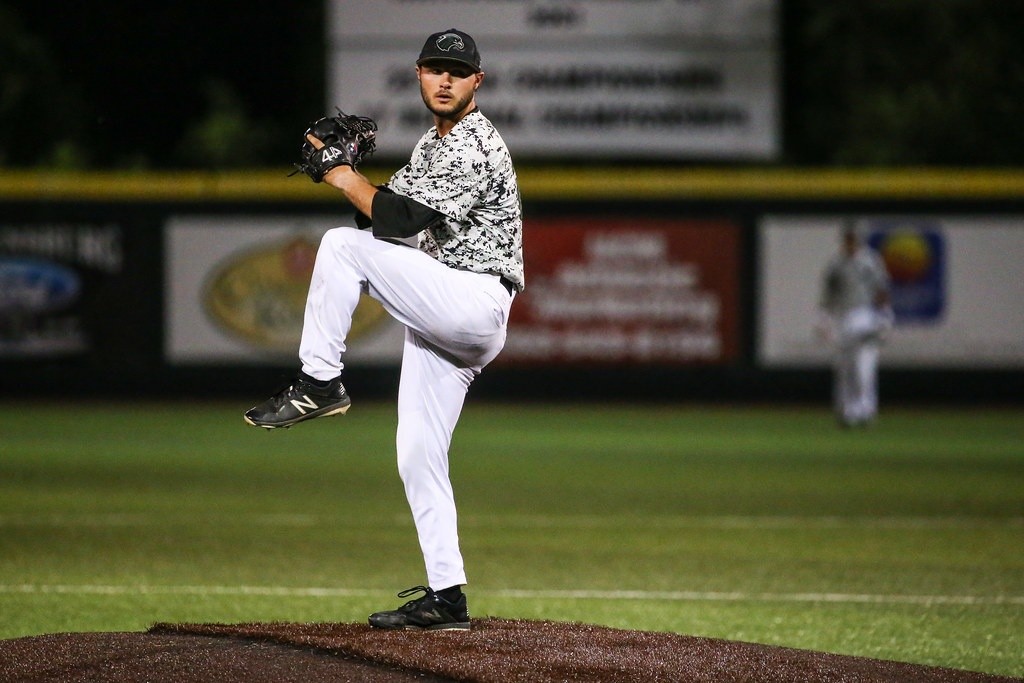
[296,116,376,183]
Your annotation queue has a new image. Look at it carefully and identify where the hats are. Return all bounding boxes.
[416,29,481,72]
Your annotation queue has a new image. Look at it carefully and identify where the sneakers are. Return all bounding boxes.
[244,373,351,431]
[368,586,470,631]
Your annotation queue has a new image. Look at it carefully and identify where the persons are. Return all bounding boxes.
[244,25,524,632]
[812,214,896,434]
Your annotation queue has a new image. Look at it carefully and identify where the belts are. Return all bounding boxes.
[500,276,512,296]
